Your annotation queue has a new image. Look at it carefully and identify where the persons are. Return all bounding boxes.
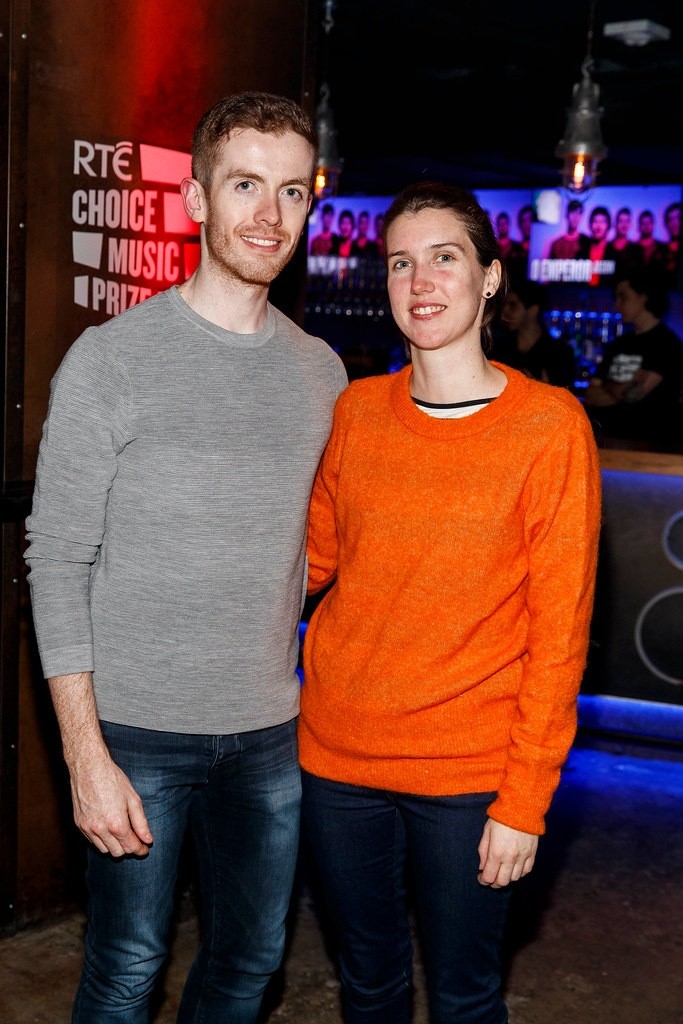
[581,267,683,454]
[486,279,576,399]
[20,90,350,1023]
[295,180,604,1024]
[309,201,683,287]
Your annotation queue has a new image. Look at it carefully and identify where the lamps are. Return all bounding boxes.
[559,0,606,206]
[312,82,346,196]
